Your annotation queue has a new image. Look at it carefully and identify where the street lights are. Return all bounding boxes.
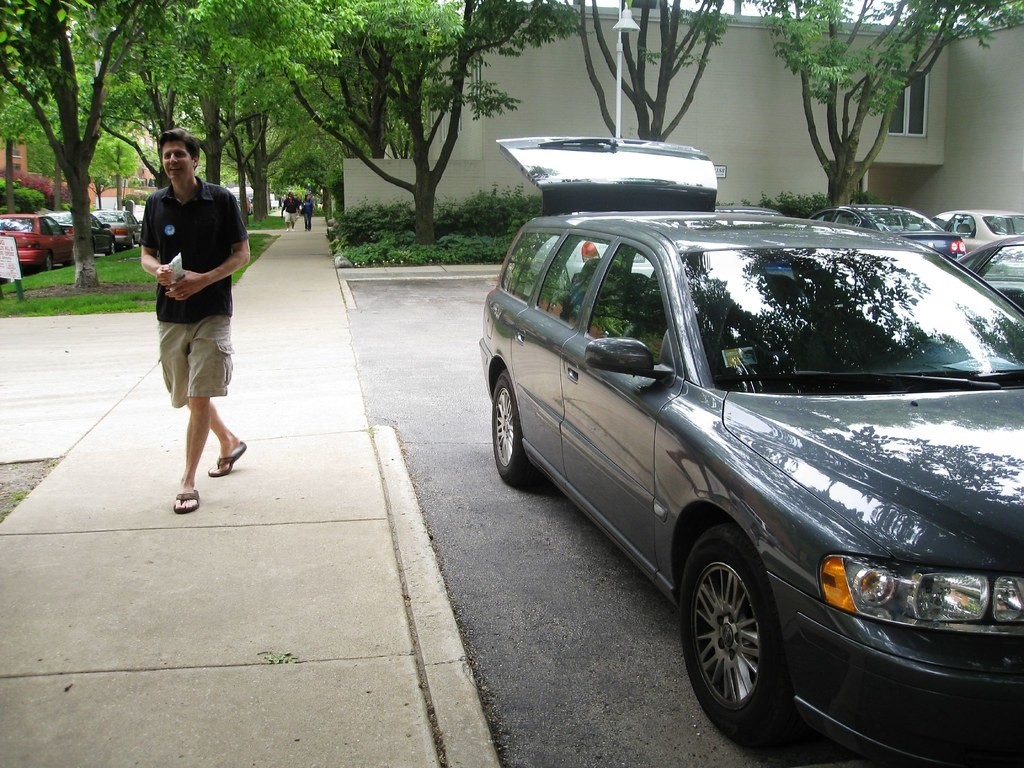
[611,4,642,137]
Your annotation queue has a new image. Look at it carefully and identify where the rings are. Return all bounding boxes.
[181,293,184,297]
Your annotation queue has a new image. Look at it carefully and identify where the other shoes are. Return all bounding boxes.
[292,229,294,231]
[286,228,289,232]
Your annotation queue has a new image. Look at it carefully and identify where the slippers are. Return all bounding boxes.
[173,490,199,513]
[208,441,247,477]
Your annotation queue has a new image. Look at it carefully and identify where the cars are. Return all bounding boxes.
[0,187,280,275]
[477,138,1024,768]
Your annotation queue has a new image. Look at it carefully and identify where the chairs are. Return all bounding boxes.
[991,222,1008,234]
[956,224,971,233]
[569,259,624,321]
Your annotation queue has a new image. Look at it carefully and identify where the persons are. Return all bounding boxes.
[138,128,250,515]
[281,192,315,232]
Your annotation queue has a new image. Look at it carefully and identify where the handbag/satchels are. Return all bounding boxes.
[301,208,305,214]
